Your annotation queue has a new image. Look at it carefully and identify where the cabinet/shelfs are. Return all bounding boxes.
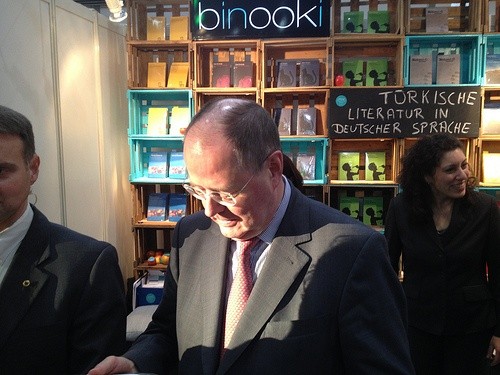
[125,0,500,279]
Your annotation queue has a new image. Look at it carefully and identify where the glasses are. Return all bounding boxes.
[182,155,268,206]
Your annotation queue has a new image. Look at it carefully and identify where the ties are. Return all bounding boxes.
[220,236,261,364]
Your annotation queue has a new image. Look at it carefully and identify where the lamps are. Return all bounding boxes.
[105,0,127,22]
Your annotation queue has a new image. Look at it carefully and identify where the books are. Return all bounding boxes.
[144,3,500,229]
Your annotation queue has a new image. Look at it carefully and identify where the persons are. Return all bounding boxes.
[0,105,126,375]
[87,98,414,374]
[281,152,304,194]
[384,133,500,375]
